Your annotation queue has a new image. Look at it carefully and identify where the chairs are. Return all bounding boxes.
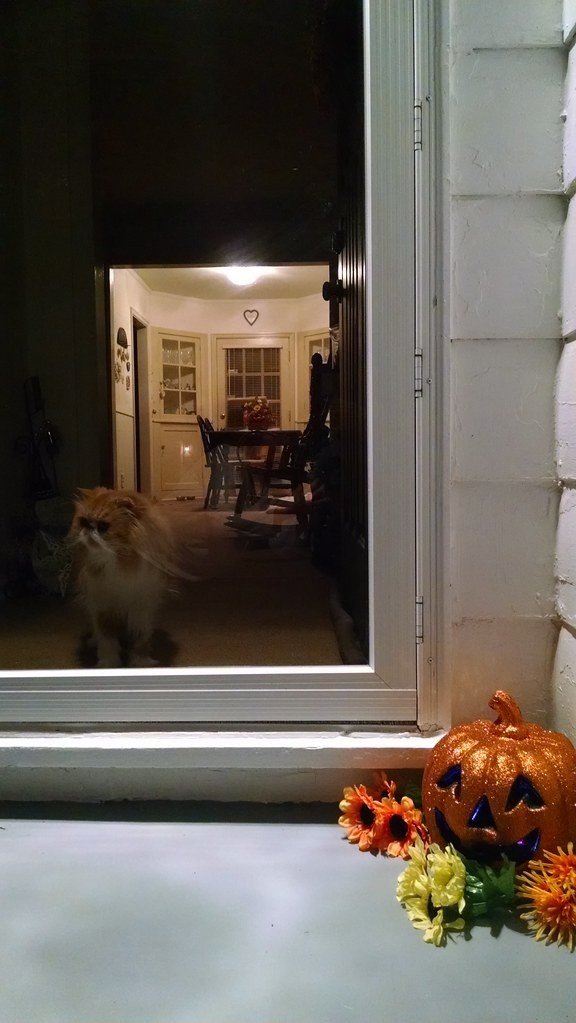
[197,414,252,511]
[205,418,243,504]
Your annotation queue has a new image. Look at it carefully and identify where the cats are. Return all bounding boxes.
[63,487,181,669]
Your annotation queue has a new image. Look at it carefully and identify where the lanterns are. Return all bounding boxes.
[422,690,576,874]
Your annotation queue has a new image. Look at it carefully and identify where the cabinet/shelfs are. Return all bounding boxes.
[151,326,209,501]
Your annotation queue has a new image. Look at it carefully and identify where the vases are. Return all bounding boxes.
[248,415,269,430]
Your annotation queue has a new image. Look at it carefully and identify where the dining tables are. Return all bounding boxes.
[206,429,304,510]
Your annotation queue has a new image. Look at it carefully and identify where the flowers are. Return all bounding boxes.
[529,841,576,901]
[338,785,382,852]
[426,841,468,915]
[396,832,429,903]
[243,394,271,416]
[513,857,576,954]
[405,886,466,948]
[371,794,432,859]
[365,769,398,803]
